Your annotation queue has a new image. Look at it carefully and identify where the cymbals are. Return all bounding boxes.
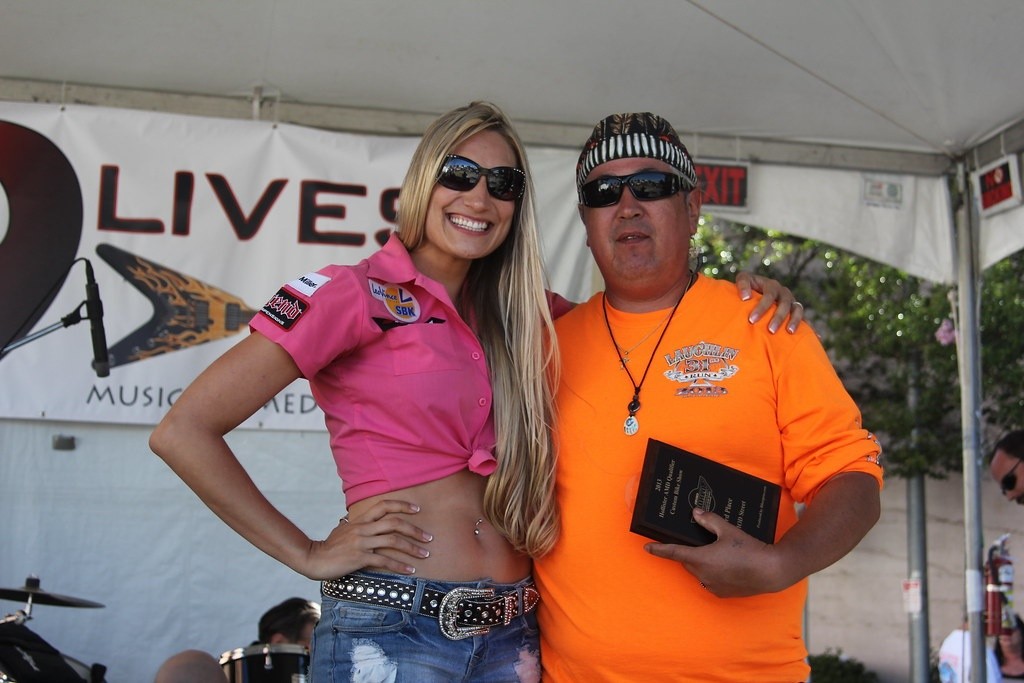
[1,582,106,611]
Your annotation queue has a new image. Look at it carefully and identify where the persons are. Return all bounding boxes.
[989,428,1024,506]
[150,99,822,683]
[154,649,230,683]
[938,609,1003,683]
[994,614,1024,683]
[250,597,322,651]
[531,112,883,683]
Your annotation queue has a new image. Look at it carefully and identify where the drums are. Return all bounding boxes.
[218,642,311,683]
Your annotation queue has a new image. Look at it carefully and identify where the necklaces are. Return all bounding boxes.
[602,270,694,436]
[603,270,693,369]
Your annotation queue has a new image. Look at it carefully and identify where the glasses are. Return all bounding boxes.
[1000,459,1020,496]
[436,153,526,202]
[580,170,691,208]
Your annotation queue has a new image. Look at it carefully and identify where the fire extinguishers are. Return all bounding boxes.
[983,533,1018,636]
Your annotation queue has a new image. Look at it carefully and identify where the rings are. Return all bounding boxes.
[699,581,706,590]
[792,302,804,311]
[340,516,349,523]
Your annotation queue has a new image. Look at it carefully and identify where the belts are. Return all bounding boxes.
[321,574,539,641]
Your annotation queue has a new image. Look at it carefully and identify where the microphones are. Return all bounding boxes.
[85,260,110,378]
